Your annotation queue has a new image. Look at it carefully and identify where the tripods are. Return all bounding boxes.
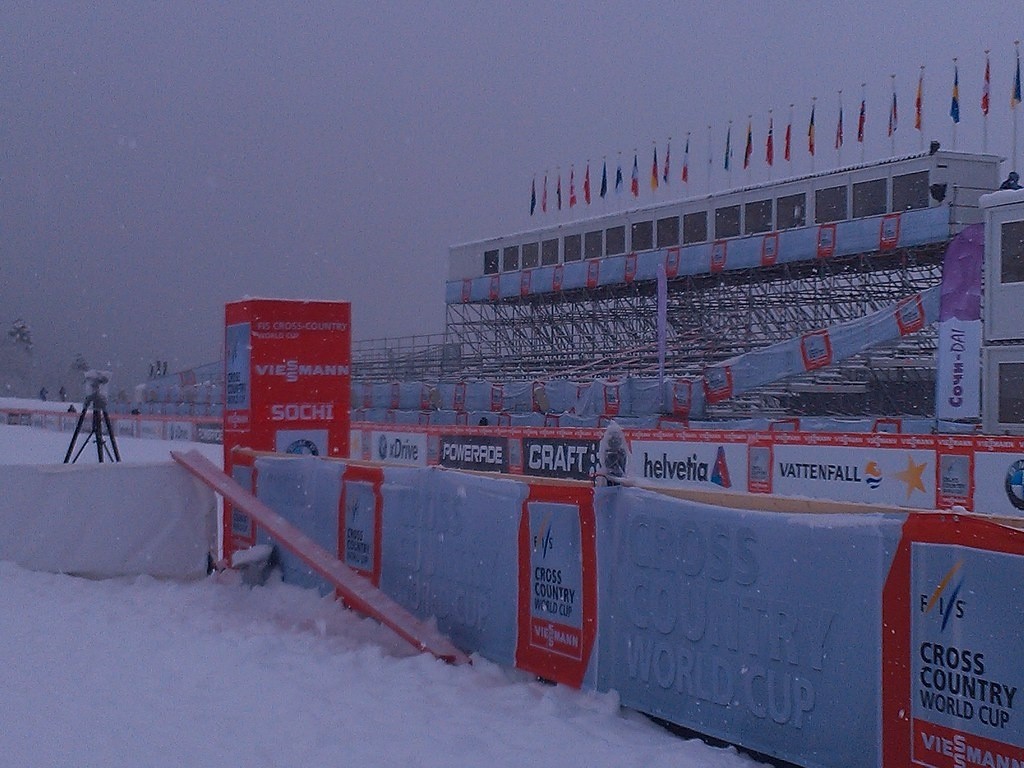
[64,372,121,464]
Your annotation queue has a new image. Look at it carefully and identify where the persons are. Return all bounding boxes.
[39,387,48,402]
[60,387,66,402]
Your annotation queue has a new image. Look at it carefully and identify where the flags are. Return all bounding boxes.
[765,119,775,167]
[651,148,659,190]
[835,108,844,149]
[723,128,729,170]
[856,100,867,143]
[981,61,990,117]
[888,92,898,139]
[530,175,562,216]
[949,68,960,124]
[808,109,815,157]
[1010,54,1022,108]
[914,78,922,132]
[631,155,639,198]
[744,123,753,170]
[569,157,624,208]
[663,145,670,183]
[682,133,713,183]
[784,124,791,162]
[937,223,984,419]
